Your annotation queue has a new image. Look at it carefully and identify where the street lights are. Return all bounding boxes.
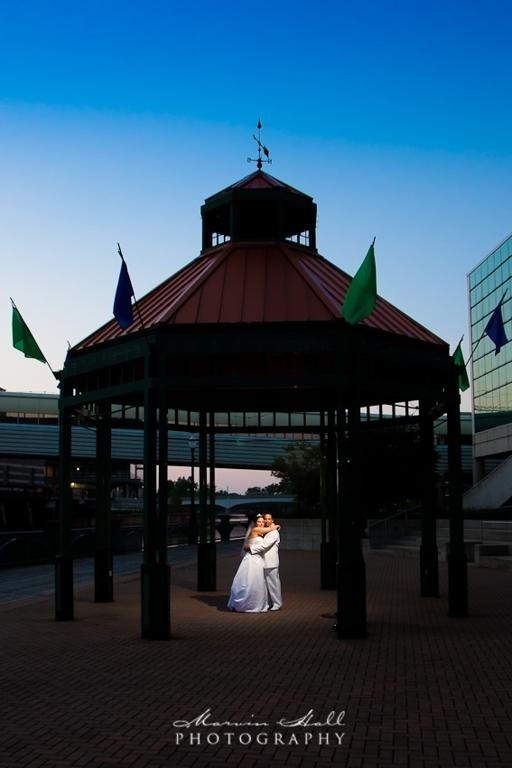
[185,434,200,546]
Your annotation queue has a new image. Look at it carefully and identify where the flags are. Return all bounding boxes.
[113,251,134,328]
[485,304,508,356]
[12,307,47,362]
[340,243,378,326]
[452,346,470,392]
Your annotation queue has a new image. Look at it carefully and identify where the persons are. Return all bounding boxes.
[243,513,283,611]
[227,514,282,614]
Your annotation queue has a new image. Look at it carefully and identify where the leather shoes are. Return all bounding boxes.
[270,603,280,611]
